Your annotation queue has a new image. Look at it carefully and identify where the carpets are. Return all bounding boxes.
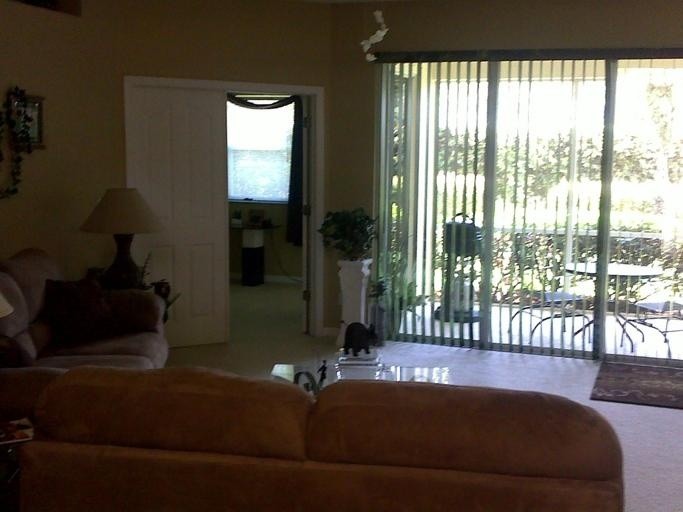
[589,354,682,413]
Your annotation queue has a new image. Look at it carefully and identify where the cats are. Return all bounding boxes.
[339,319,379,357]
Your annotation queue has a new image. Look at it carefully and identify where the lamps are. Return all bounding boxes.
[0,290,14,321]
[78,185,160,290]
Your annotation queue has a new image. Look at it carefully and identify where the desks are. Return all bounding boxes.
[561,261,666,352]
[0,411,39,512]
[227,221,274,287]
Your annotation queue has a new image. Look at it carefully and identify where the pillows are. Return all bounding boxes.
[41,271,117,347]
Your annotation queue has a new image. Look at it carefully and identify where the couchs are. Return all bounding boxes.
[0,243,171,415]
[18,362,627,512]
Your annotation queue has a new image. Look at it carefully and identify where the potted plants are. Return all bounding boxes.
[231,209,244,224]
[316,205,380,349]
[363,276,388,348]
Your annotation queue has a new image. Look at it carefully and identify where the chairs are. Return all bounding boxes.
[500,231,593,345]
[618,261,683,361]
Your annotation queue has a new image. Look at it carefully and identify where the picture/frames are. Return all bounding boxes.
[4,93,46,153]
